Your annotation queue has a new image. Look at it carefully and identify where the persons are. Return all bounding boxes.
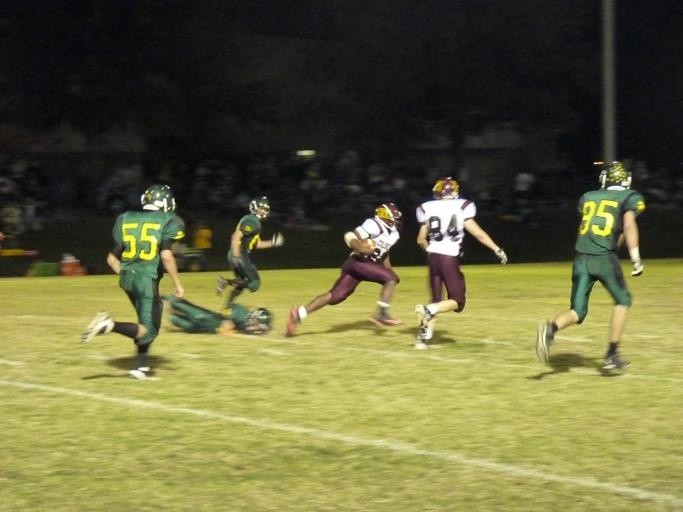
[536,160,647,376]
[286,200,407,335]
[217,196,288,308]
[82,183,188,380]
[0,95,682,239]
[414,174,508,350]
[159,293,271,336]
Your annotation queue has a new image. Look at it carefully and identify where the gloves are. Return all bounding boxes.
[630,246,643,277]
[493,247,507,265]
[271,232,285,246]
[232,255,245,269]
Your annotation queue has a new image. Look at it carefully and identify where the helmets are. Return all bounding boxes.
[249,197,270,220]
[433,178,459,200]
[245,308,270,334]
[598,161,632,190]
[375,203,402,227]
[141,184,176,213]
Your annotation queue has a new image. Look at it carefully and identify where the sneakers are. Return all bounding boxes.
[603,356,632,370]
[288,304,302,337]
[79,311,113,345]
[416,304,434,351]
[217,275,228,296]
[130,368,162,381]
[536,320,552,365]
[370,306,403,328]
